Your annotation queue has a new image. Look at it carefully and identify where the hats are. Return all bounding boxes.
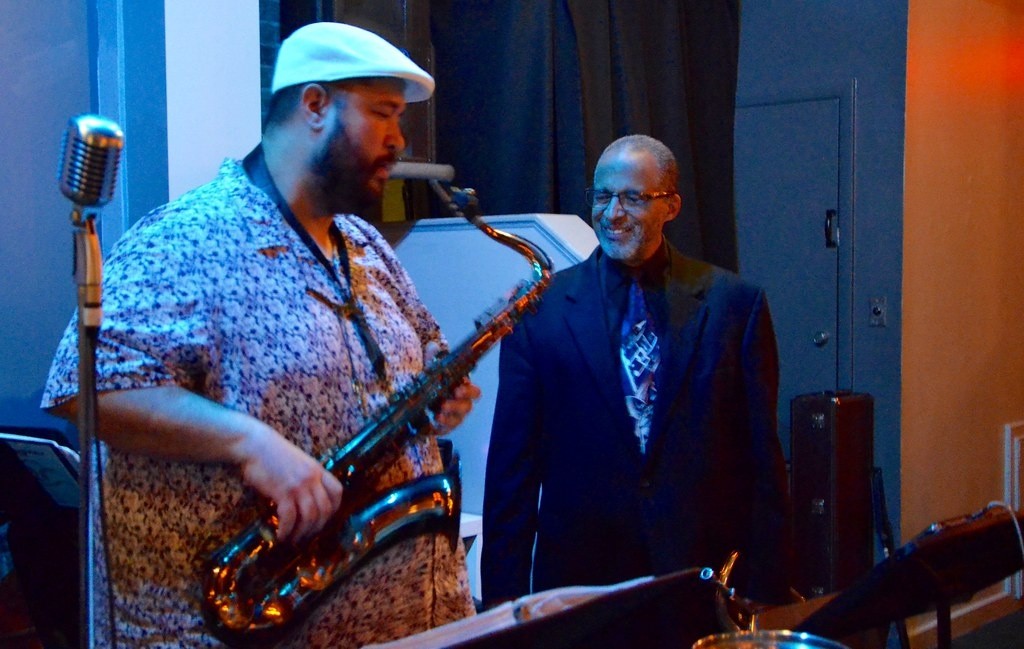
[272,19,435,103]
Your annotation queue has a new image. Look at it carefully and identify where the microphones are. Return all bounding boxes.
[58,115,125,339]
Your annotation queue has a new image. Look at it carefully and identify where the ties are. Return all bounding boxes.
[621,268,661,456]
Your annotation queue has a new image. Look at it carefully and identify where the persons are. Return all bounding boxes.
[481,135,784,649]
[42,22,481,649]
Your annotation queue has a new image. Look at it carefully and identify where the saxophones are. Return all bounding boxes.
[196,183,555,648]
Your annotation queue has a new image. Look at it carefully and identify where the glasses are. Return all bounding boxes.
[584,186,678,213]
